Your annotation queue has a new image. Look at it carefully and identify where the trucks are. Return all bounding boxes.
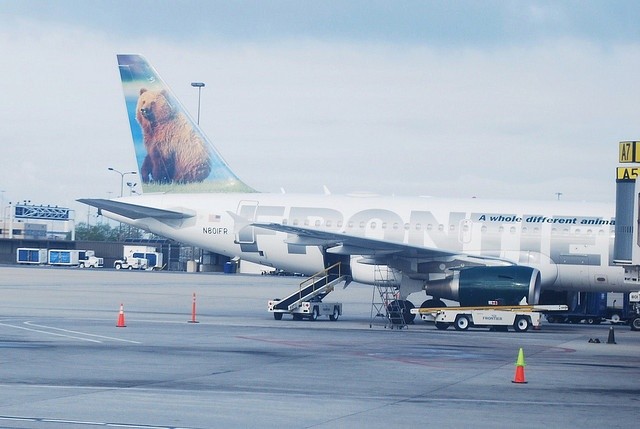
[15,247,103,268]
[114,256,148,270]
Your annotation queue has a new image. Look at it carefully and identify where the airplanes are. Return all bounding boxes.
[75,55,640,331]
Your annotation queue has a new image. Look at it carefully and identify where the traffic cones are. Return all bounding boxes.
[188,291,199,324]
[512,348,527,384]
[607,326,617,344]
[116,303,127,327]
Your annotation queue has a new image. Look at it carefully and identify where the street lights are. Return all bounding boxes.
[126,181,136,236]
[190,82,205,125]
[108,168,137,241]
